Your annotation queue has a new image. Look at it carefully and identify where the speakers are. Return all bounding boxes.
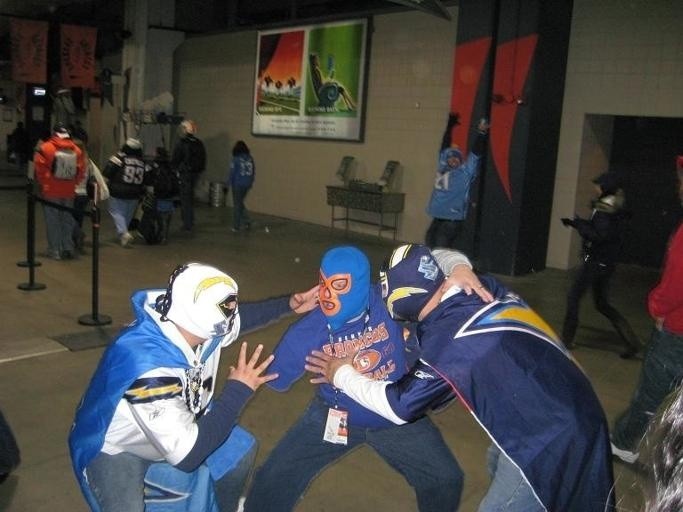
[379,160,401,192]
[335,156,357,188]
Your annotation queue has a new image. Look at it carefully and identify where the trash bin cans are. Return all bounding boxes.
[209,182,225,207]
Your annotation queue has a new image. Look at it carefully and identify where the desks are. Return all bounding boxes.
[326,185,406,248]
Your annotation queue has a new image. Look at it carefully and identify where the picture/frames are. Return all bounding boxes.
[250,15,372,142]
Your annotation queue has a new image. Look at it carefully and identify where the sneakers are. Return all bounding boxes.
[121,232,133,248]
[610,437,638,464]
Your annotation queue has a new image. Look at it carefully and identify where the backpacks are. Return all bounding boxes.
[52,150,77,180]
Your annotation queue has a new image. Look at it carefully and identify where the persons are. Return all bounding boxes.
[339,417,348,427]
[559,174,646,359]
[424,111,493,249]
[11,120,206,261]
[225,140,257,233]
[100,68,113,107]
[312,55,358,111]
[305,242,615,512]
[609,157,683,465]
[67,262,320,512]
[243,246,496,512]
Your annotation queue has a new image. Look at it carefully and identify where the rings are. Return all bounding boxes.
[480,286,484,289]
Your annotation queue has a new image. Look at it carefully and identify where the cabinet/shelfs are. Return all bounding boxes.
[129,108,187,163]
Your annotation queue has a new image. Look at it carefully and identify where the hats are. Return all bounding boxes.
[591,174,615,186]
[53,122,70,139]
[444,148,461,161]
[181,119,199,134]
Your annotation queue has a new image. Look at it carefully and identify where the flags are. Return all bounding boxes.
[61,23,97,88]
[10,17,47,85]
